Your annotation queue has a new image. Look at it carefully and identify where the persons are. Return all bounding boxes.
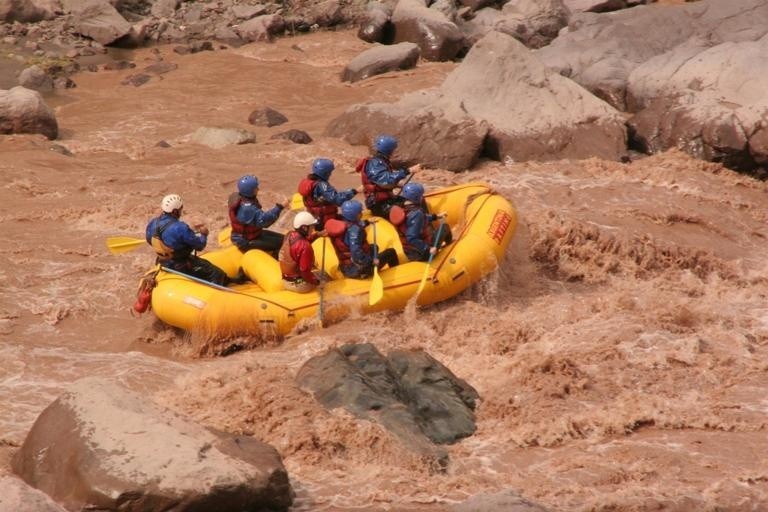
[145,195,229,287]
[325,200,399,280]
[297,158,358,225]
[279,212,332,294]
[382,182,452,262]
[355,136,409,220]
[228,175,290,254]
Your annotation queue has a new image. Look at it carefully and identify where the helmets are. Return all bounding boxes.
[402,183,425,206]
[161,194,183,214]
[312,158,334,181]
[238,176,259,198]
[293,212,318,231]
[341,200,363,223]
[376,136,397,158]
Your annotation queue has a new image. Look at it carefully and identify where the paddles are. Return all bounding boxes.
[368,223,383,305]
[217,198,291,245]
[418,217,446,293]
[106,223,207,255]
[288,185,361,208]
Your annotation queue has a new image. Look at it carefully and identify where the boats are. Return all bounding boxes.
[137,181,516,334]
